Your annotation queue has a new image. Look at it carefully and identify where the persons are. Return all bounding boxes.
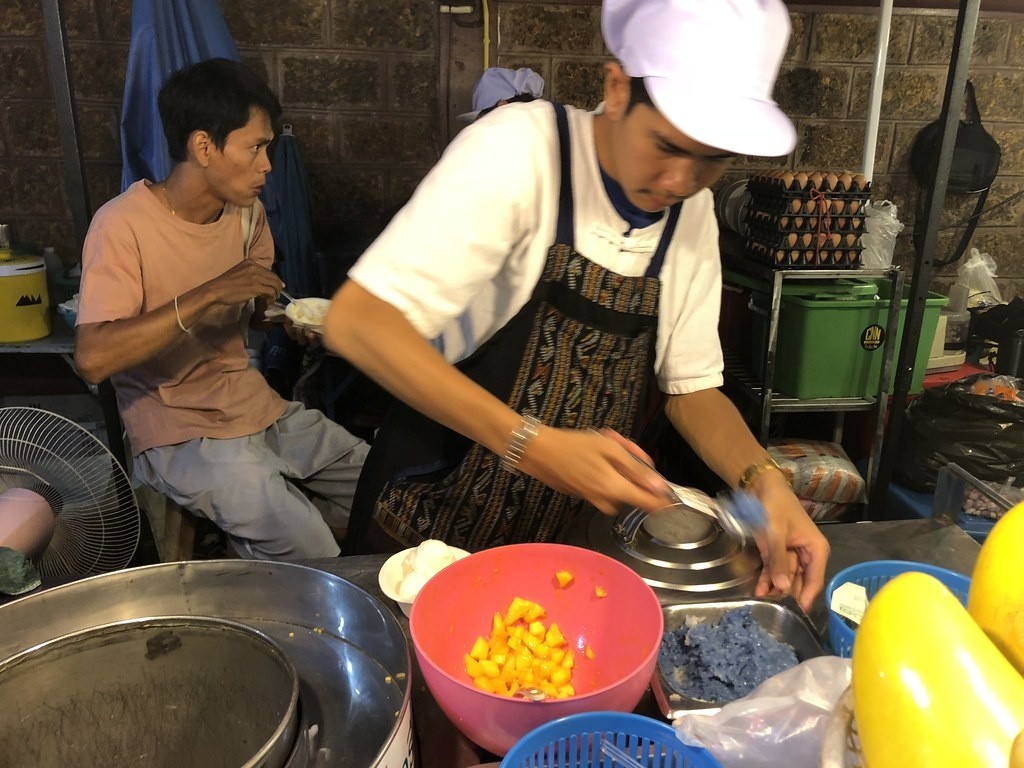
[74,57,372,560]
[322,0,831,618]
[457,65,547,123]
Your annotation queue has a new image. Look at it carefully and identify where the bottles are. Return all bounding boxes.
[42,247,65,312]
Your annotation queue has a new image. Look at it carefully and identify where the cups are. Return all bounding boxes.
[0,223,13,261]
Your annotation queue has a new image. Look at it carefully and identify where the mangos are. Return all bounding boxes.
[850,496,1024,768]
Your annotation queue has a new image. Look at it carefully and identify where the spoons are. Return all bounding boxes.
[281,290,313,318]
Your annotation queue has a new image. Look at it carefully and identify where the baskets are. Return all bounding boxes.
[499,711,723,768]
[825,559,971,659]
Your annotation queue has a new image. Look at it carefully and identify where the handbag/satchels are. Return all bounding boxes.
[912,79,1001,194]
[957,247,1002,309]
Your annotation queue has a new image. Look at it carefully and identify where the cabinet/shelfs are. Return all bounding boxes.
[718,239,904,521]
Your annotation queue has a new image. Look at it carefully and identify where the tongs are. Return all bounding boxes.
[577,421,769,540]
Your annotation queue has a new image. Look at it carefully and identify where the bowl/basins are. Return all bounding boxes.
[410,541,664,759]
[56,305,77,331]
[378,546,472,619]
[285,298,332,329]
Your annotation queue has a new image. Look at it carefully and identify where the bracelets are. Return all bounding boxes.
[739,458,787,490]
[175,296,193,335]
[500,411,543,476]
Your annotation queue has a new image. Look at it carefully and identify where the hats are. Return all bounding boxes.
[456,67,545,122]
[603,0,799,157]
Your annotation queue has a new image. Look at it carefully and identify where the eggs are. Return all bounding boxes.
[745,169,867,263]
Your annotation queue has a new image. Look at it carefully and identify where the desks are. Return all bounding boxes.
[0,313,132,514]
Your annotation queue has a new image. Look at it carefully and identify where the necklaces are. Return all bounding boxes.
[161,178,174,215]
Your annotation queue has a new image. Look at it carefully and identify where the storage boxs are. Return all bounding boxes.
[722,267,950,400]
[0,395,132,512]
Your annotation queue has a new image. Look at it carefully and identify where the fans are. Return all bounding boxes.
[0,406,140,596]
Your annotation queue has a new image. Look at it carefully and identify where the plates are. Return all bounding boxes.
[715,178,752,238]
